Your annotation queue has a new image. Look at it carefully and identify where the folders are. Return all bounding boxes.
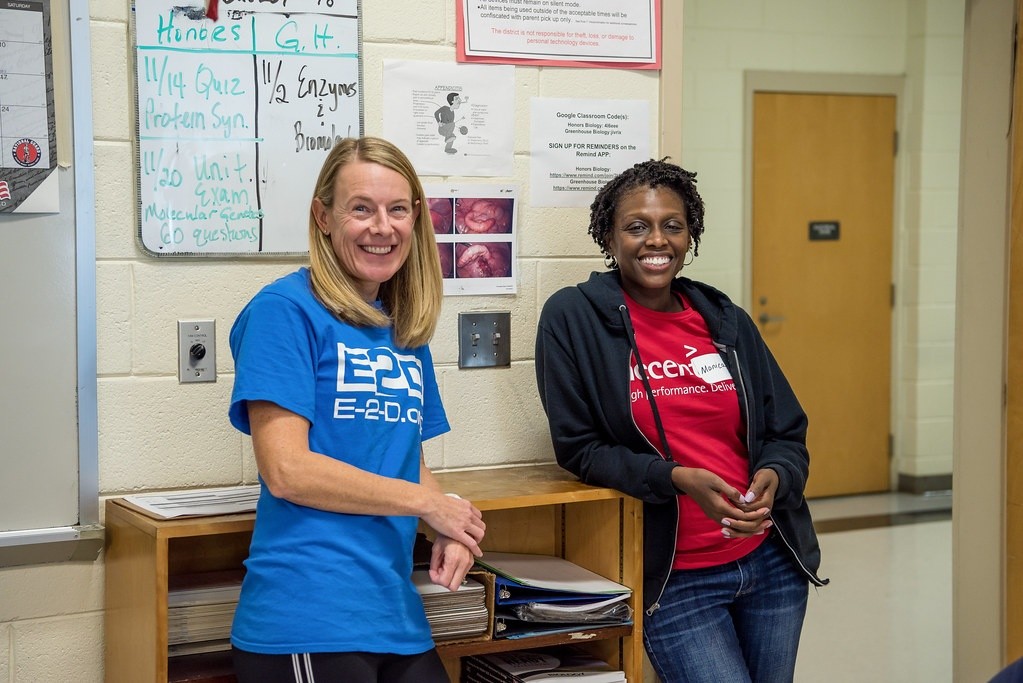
[495,614,634,641]
[497,579,632,607]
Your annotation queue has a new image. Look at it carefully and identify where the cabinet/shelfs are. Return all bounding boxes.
[104,465,643,683]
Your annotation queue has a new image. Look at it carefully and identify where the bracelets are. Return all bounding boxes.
[445,493,461,499]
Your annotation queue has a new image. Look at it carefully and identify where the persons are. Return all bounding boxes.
[534,155,830,683]
[227,137,487,683]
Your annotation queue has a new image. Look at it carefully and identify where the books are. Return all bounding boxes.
[473,554,633,594]
[461,643,628,683]
